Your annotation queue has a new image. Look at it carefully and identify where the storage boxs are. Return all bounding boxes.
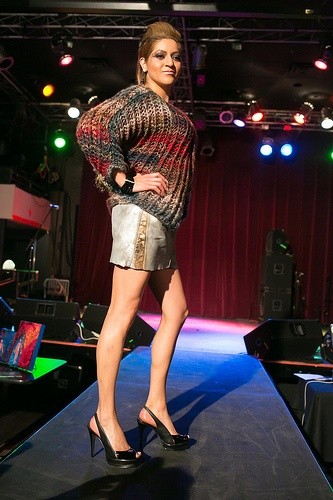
[261,255,292,290]
[259,288,291,320]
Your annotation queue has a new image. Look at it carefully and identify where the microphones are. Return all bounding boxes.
[49,204,59,209]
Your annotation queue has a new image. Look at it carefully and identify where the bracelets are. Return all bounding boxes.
[121,174,135,195]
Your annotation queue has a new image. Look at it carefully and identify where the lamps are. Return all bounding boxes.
[1,1,333,173]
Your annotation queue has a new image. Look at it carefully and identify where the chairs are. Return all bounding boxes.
[293,381,333,462]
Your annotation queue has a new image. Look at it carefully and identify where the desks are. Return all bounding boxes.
[0,357,67,384]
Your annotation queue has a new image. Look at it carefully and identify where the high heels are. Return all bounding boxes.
[87,412,144,469]
[137,405,191,451]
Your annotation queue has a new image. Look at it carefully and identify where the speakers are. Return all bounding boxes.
[258,256,292,323]
[81,304,156,346]
[320,324,333,362]
[0,297,13,328]
[243,319,323,360]
[14,297,81,342]
[265,230,287,256]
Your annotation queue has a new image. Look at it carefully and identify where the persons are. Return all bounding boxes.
[76,21,198,469]
